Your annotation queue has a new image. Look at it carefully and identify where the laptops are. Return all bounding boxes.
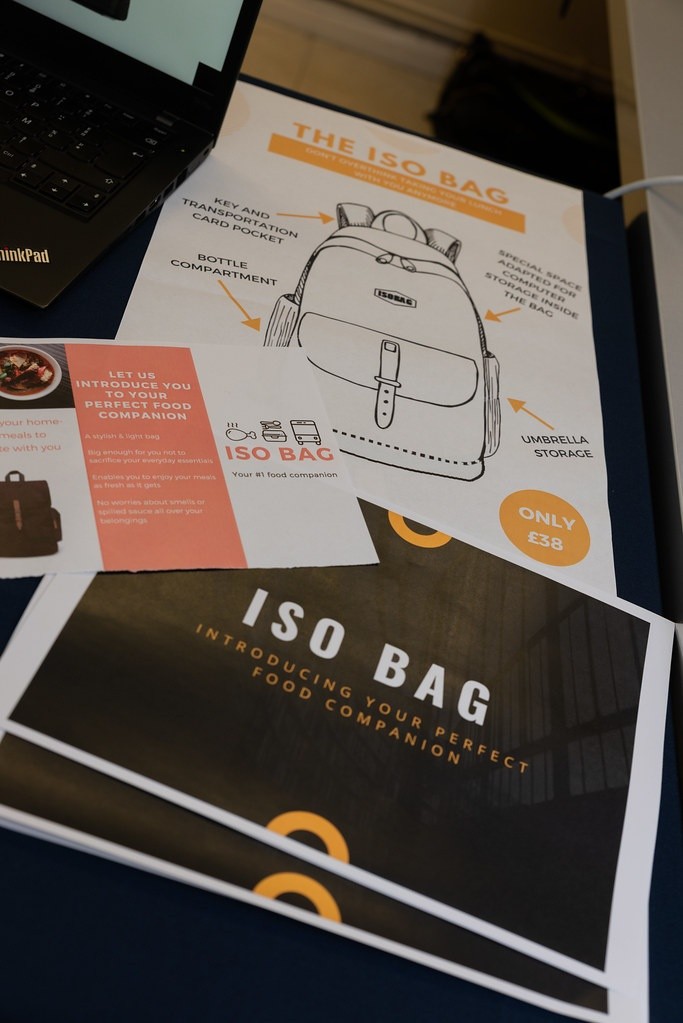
[1,0,263,311]
